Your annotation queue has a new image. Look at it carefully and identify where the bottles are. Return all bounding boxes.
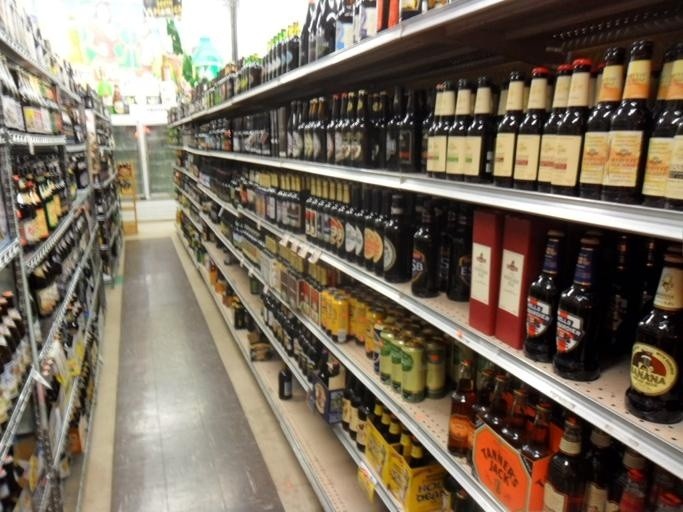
[1,1,124,511]
[111,84,123,113]
[166,2,682,511]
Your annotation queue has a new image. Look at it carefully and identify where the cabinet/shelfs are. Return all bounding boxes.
[167,1,683,511]
[0,36,125,509]
